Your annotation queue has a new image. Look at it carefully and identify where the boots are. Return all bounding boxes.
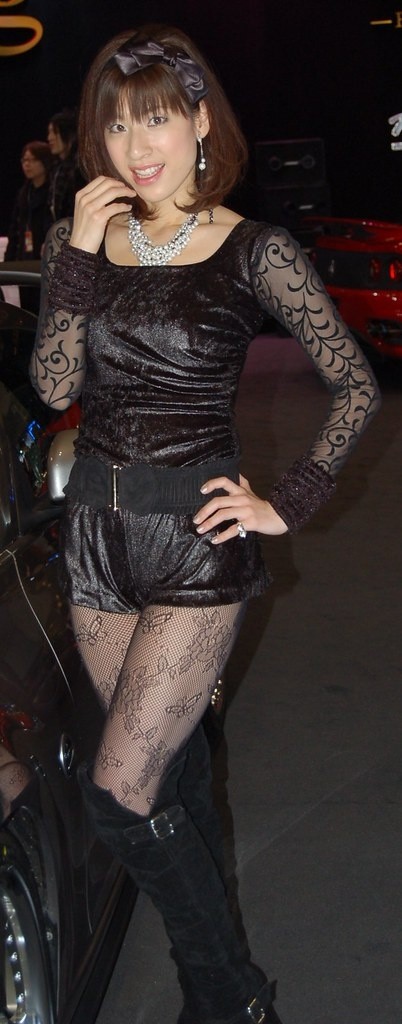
[77,748,283,1024]
[175,722,269,1024]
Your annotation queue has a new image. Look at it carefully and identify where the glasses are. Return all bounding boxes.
[21,158,36,163]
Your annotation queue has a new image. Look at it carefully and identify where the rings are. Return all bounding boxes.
[237,522,247,539]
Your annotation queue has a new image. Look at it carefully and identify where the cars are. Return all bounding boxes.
[1,254,142,1021]
[283,213,402,383]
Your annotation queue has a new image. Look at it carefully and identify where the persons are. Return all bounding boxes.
[0,744,67,930]
[15,109,78,259]
[30,21,383,1024]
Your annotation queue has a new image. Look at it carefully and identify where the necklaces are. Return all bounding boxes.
[127,213,198,266]
